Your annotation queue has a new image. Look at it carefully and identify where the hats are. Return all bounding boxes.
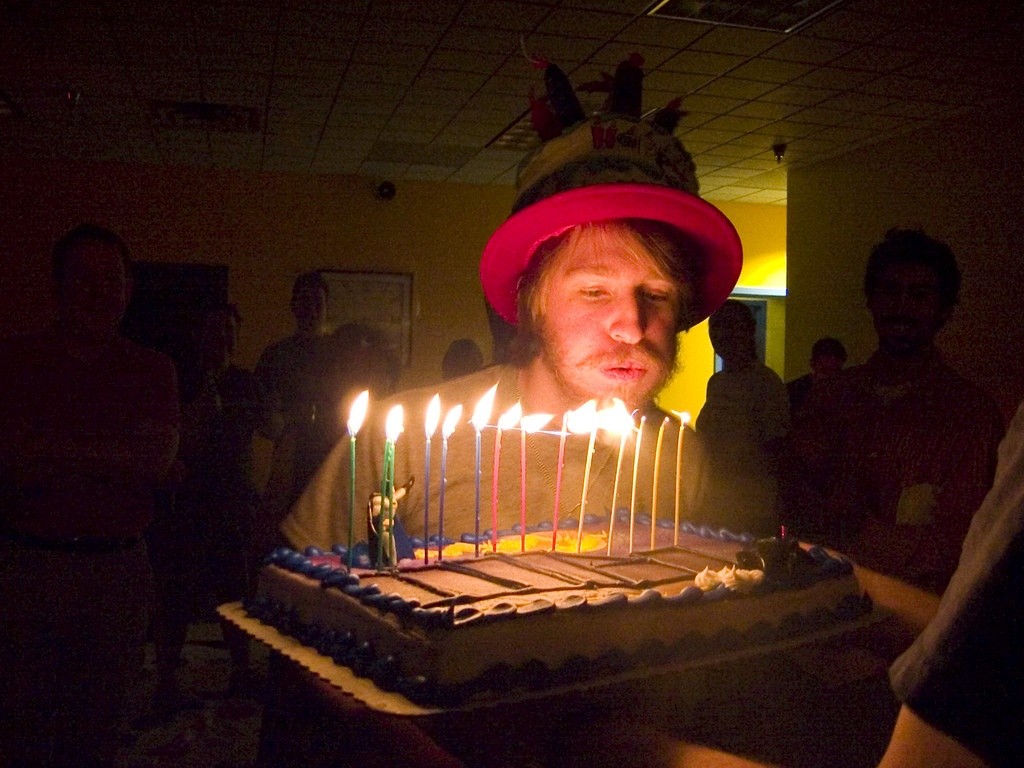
[480,52,743,334]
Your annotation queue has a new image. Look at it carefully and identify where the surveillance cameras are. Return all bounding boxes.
[374,176,397,202]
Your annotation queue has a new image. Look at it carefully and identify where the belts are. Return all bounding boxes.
[0,524,143,554]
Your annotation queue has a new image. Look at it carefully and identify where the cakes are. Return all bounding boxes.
[241,513,873,707]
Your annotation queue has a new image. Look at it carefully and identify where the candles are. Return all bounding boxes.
[346,388,690,574]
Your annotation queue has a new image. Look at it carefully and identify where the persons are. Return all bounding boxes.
[253,268,399,543]
[444,339,481,382]
[787,339,848,406]
[366,476,415,567]
[697,296,798,540]
[265,58,744,558]
[788,225,1004,602]
[556,400,1024,768]
[147,304,285,700]
[0,224,179,768]
